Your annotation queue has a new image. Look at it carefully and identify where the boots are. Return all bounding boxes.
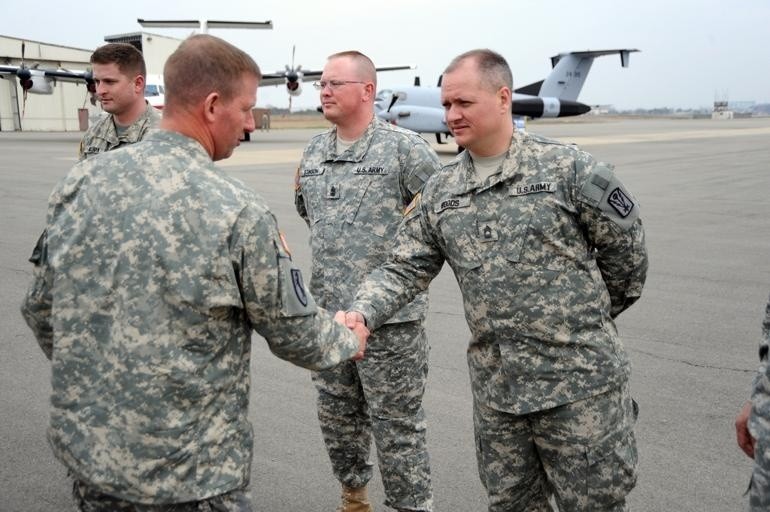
[333,485,373,511]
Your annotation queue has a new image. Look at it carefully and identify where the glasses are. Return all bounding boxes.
[312,79,367,89]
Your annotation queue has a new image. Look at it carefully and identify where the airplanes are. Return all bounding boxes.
[374,49,641,145]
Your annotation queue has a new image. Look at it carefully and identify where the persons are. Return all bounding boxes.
[21,33,370,512]
[261,112,268,133]
[296,50,442,511]
[76,41,163,166]
[346,47,649,512]
[735,303,769,512]
